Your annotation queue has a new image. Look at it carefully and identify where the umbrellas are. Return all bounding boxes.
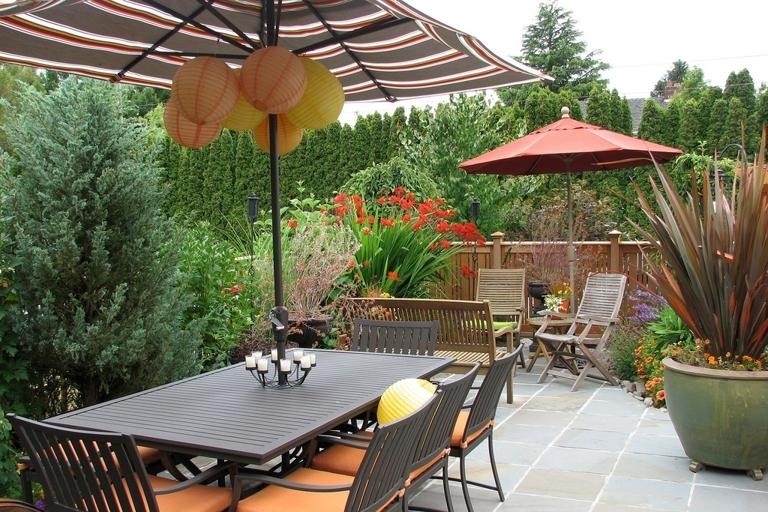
[458,106,682,353]
[0,0,557,475]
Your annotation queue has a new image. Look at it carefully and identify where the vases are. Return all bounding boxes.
[527,281,552,315]
[549,298,570,320]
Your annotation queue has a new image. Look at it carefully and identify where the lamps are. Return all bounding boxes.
[243,347,318,390]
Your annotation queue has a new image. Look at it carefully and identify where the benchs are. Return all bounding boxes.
[345,295,515,405]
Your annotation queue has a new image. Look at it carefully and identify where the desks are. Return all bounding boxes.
[525,316,580,376]
[37,349,460,512]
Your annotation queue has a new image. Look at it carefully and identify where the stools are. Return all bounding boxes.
[15,436,203,505]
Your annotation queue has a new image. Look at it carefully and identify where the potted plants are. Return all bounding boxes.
[611,117,768,481]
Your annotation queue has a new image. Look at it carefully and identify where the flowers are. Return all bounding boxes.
[659,335,767,372]
[541,277,573,310]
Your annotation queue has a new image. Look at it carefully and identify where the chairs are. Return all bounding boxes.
[307,359,485,512]
[533,270,628,394]
[399,340,526,512]
[459,266,527,375]
[340,317,442,434]
[235,386,443,512]
[4,411,245,512]
